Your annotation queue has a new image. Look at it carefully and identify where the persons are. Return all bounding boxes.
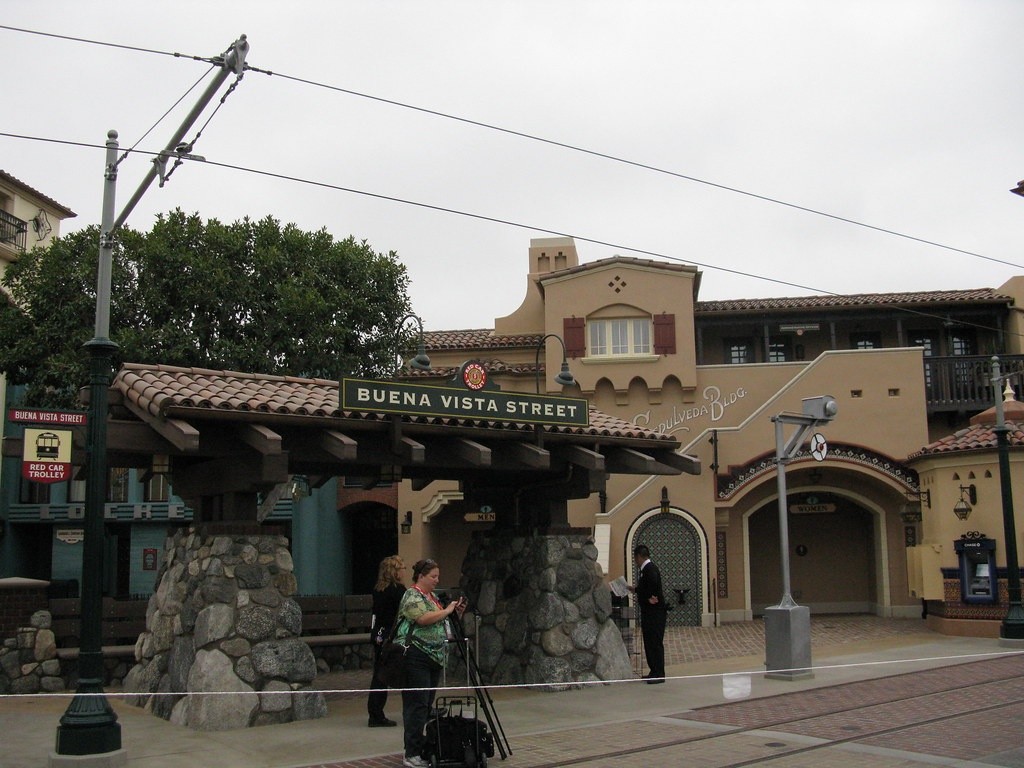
[391,559,467,768]
[367,555,407,727]
[628,544,668,685]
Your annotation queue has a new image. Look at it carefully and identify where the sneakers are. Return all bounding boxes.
[403,749,406,759]
[427,707,448,720]
[403,753,428,768]
[369,717,397,727]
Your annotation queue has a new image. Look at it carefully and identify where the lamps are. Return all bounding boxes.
[944,313,955,327]
[534,332,577,393]
[290,472,312,502]
[900,489,932,525]
[400,510,414,535]
[387,313,433,382]
[954,482,977,522]
[659,486,671,516]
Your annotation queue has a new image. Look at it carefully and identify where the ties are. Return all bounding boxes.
[640,569,642,578]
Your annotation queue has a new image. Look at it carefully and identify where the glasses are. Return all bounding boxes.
[398,567,406,571]
[419,559,434,572]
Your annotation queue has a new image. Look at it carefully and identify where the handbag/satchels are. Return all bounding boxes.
[418,701,495,761]
[370,624,399,648]
[378,617,417,689]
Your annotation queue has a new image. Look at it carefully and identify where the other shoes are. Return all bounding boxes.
[643,676,649,678]
[647,679,665,684]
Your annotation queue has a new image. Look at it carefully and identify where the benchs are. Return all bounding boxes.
[55,592,376,663]
